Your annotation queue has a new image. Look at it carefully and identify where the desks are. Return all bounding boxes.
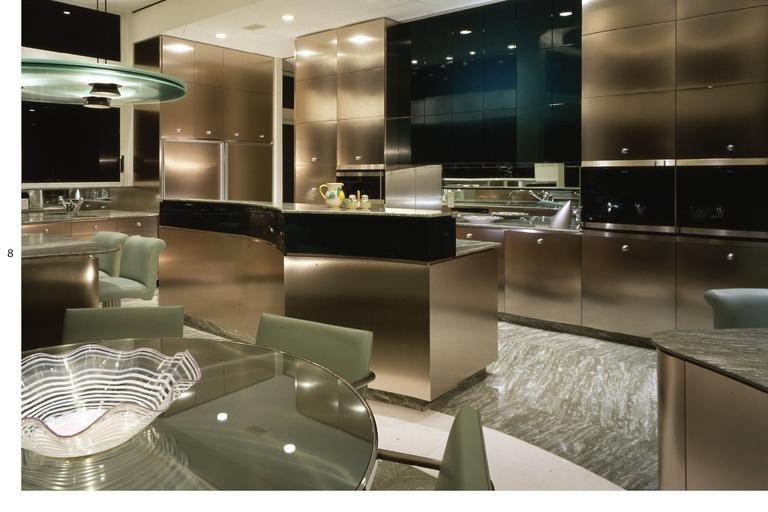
[22,235,120,350]
[21,336,378,491]
[652,328,768,490]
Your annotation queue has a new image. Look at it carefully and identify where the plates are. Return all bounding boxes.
[21,346,201,456]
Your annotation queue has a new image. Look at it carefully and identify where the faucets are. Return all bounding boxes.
[75,198,85,213]
[58,196,67,211]
[529,190,547,202]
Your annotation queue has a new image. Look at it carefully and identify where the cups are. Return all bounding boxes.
[28,190,42,208]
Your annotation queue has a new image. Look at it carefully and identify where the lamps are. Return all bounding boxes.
[81,0,111,108]
[90,0,121,97]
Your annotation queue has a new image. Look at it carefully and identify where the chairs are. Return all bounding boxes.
[60,307,183,345]
[368,404,494,491]
[256,312,376,402]
[705,286,768,330]
[95,230,128,279]
[100,234,167,307]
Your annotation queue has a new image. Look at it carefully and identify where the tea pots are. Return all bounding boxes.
[318,183,345,207]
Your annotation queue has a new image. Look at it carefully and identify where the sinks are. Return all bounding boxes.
[522,215,554,224]
[72,215,103,220]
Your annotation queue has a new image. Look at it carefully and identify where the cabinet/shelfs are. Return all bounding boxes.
[22,210,159,240]
